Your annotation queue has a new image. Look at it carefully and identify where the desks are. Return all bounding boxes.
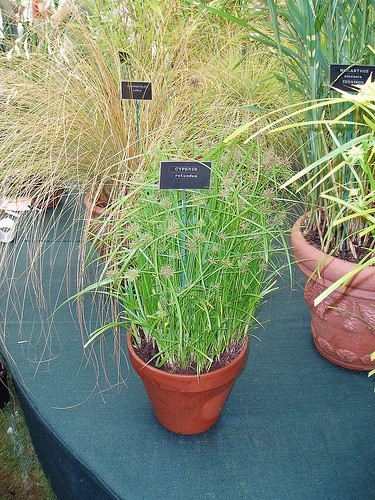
[0,160,375,500]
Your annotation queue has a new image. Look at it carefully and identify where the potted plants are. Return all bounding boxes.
[0,0,375,435]
